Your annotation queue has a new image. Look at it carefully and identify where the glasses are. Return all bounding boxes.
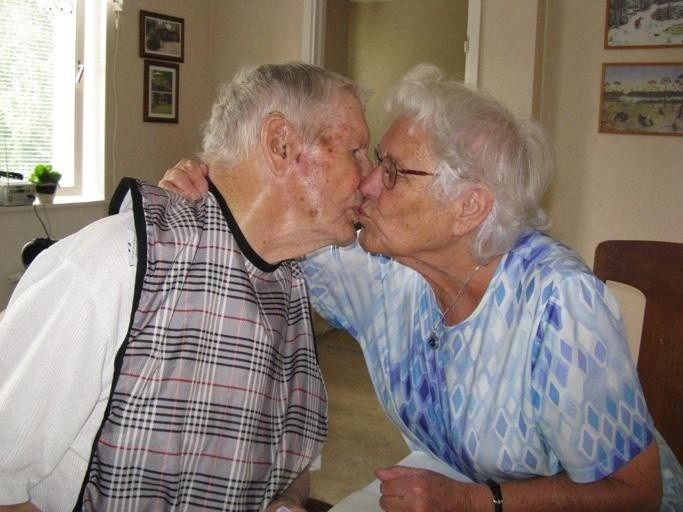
[369,147,480,191]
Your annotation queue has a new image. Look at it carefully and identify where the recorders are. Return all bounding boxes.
[0,181,37,206]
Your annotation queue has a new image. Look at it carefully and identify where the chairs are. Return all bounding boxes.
[594,238,681,465]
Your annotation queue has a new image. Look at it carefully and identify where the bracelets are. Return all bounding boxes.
[487,476,505,512]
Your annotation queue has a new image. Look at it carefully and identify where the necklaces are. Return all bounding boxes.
[422,257,484,349]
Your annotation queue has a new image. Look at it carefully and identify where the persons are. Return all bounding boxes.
[159,61,683,511]
[0,61,375,512]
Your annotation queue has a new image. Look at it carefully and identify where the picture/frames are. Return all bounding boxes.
[598,0,683,136]
[140,10,184,123]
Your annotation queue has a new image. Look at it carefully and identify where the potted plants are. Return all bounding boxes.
[28,164,62,203]
